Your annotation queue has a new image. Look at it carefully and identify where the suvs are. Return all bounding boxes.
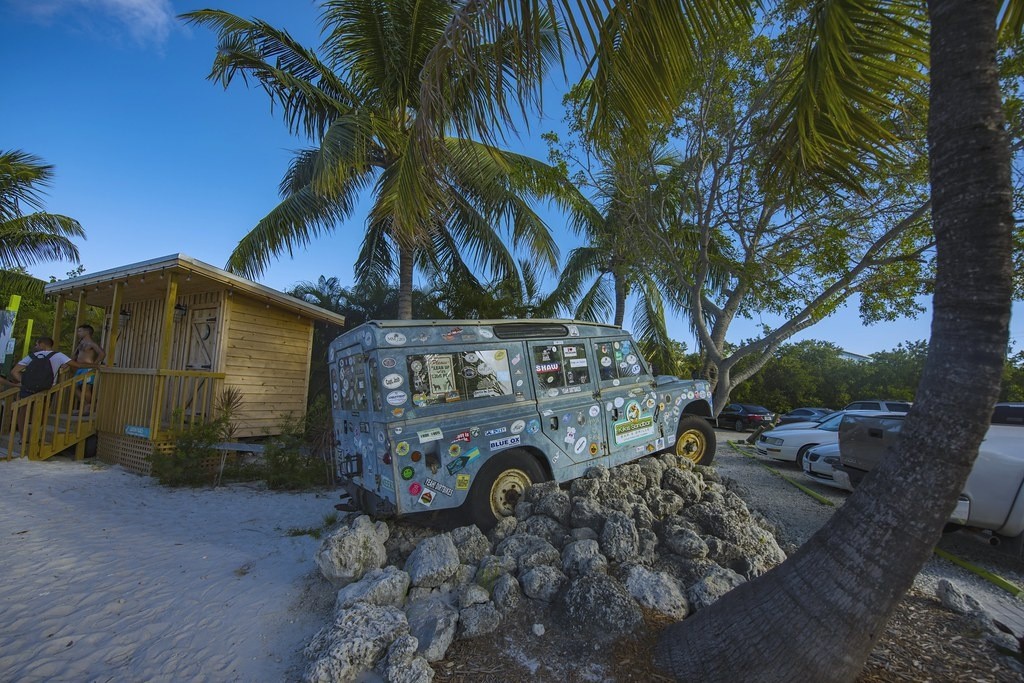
[326,317,720,536]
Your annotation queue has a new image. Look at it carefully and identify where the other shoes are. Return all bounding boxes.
[19,438,41,445]
[72,411,90,416]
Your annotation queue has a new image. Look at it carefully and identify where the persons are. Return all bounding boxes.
[71,324,105,413]
[11,336,101,445]
[0,364,22,435]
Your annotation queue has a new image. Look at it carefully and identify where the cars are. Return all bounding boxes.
[718,404,780,432]
[753,398,915,497]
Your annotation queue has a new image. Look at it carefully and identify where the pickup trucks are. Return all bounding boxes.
[832,402,1024,558]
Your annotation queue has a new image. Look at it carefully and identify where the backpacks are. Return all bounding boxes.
[21,351,57,393]
